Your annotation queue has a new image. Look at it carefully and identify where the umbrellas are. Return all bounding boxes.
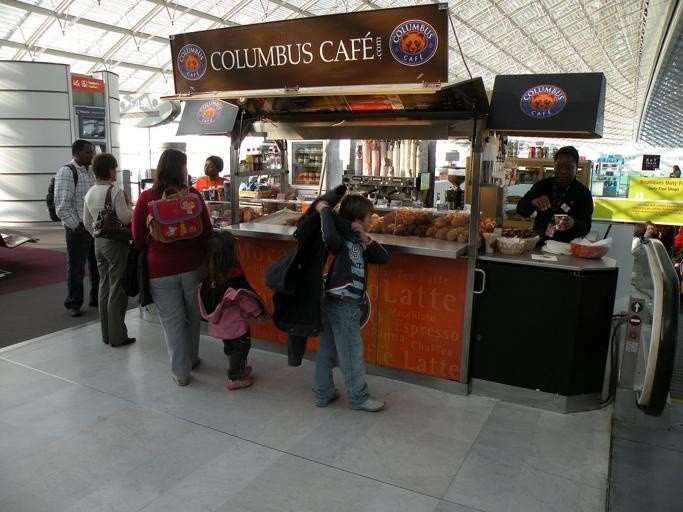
[66,306,79,317]
[109,338,137,346]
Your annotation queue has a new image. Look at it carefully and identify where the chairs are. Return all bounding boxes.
[316,392,340,406]
[171,372,190,387]
[241,365,253,376]
[352,398,386,412]
[226,378,254,390]
[191,360,202,370]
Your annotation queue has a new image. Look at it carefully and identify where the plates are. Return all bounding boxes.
[289,140,339,199]
[512,158,594,192]
[230,118,290,223]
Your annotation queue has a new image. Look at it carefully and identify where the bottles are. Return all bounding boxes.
[205,156,224,172]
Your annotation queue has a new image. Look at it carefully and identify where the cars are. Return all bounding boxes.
[223,181,231,201]
[202,189,209,201]
[554,214,567,231]
[208,189,215,200]
[217,186,224,201]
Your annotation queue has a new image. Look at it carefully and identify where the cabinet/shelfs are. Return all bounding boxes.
[208,186,215,201]
[542,147,549,159]
[215,188,218,201]
[297,155,304,165]
[309,171,315,184]
[310,155,315,166]
[535,146,542,158]
[304,153,309,165]
[528,147,535,159]
[223,180,230,201]
[303,171,309,184]
[268,146,276,170]
[256,148,264,171]
[261,146,270,171]
[252,148,258,171]
[298,172,305,184]
[315,156,321,166]
[246,149,254,172]
[203,189,209,200]
[217,185,224,201]
[550,147,558,160]
[239,160,249,172]
[274,146,282,169]
[435,192,441,206]
[315,170,320,184]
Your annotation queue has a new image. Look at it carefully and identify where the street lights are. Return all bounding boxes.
[94,184,134,243]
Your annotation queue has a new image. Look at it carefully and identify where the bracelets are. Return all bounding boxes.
[571,240,608,259]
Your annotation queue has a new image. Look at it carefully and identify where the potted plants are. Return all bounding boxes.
[92,142,107,164]
[74,104,109,143]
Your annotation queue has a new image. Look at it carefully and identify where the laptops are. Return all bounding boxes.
[369,209,431,235]
[425,212,497,242]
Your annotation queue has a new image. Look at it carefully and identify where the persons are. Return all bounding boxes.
[630,223,646,253]
[83,153,135,347]
[130,148,213,388]
[192,155,231,192]
[641,221,664,245]
[196,230,274,391]
[668,166,682,180]
[673,227,682,313]
[515,145,594,250]
[54,138,101,318]
[314,194,388,413]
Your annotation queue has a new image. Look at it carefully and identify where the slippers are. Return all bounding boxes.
[147,187,206,243]
[92,185,132,243]
[47,163,79,223]
